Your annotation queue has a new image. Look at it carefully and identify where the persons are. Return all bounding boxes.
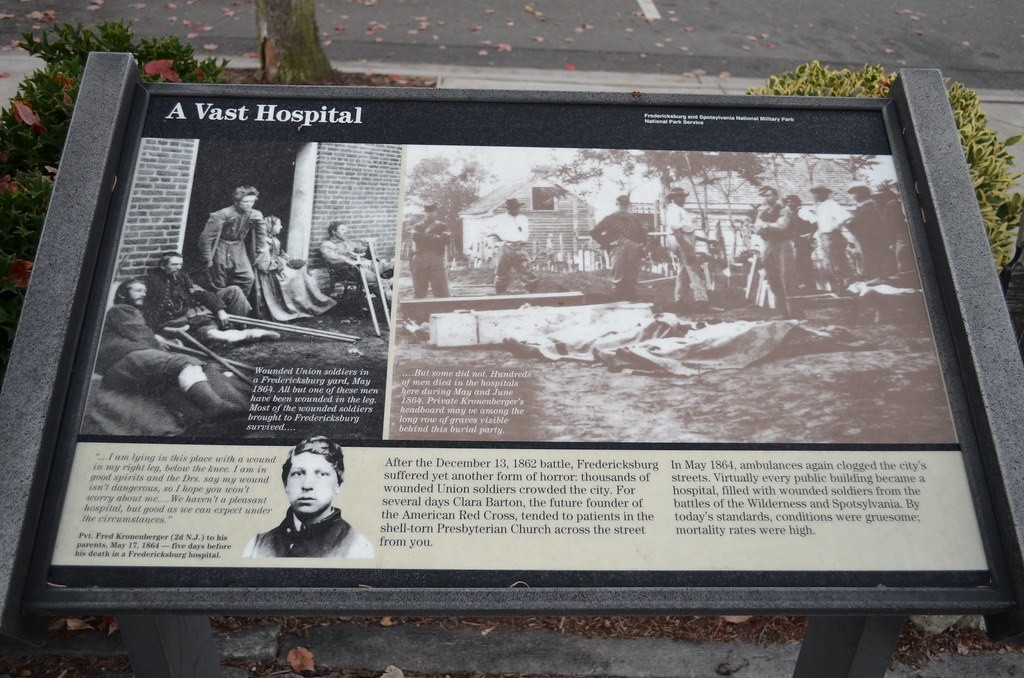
[410,182,898,323]
[244,438,374,570]
[103,181,395,422]
